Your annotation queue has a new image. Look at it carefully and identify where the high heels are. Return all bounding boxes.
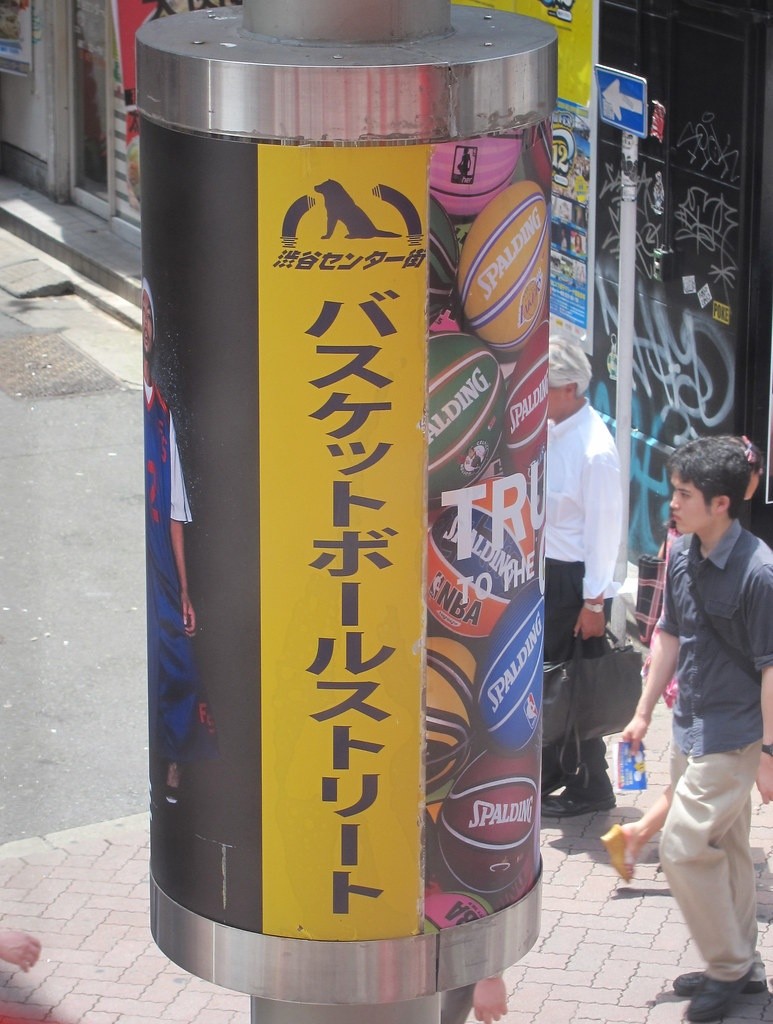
[600,824,636,884]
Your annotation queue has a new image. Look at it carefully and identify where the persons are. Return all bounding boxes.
[540,334,617,816]
[441,968,508,1024]
[0,930,43,974]
[142,278,213,812]
[602,435,773,1023]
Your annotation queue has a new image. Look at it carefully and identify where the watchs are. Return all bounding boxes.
[761,743,773,758]
[583,600,604,614]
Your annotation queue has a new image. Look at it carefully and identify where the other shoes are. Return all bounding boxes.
[164,780,180,803]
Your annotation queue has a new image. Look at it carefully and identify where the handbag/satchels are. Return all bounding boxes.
[542,627,644,776]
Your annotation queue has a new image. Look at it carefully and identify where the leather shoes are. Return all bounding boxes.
[542,788,616,817]
[542,780,563,798]
[687,971,753,1021]
[673,972,768,993]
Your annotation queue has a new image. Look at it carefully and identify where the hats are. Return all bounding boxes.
[142,278,155,343]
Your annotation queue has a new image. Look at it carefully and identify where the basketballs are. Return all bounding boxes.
[422,120,554,937]
